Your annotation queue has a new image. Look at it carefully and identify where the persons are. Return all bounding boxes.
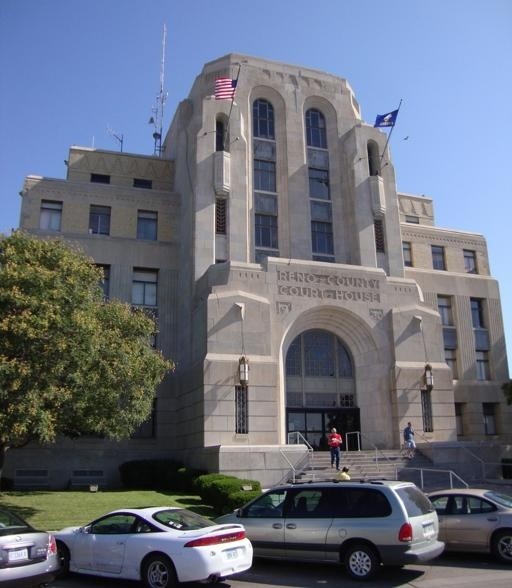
[336,465,351,480]
[404,421,416,460]
[327,427,343,471]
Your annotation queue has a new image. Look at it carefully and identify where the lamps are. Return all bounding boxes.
[425,363,434,392]
[239,356,249,387]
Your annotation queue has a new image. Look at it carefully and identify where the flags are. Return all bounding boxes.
[374,109,398,127]
[214,77,237,100]
